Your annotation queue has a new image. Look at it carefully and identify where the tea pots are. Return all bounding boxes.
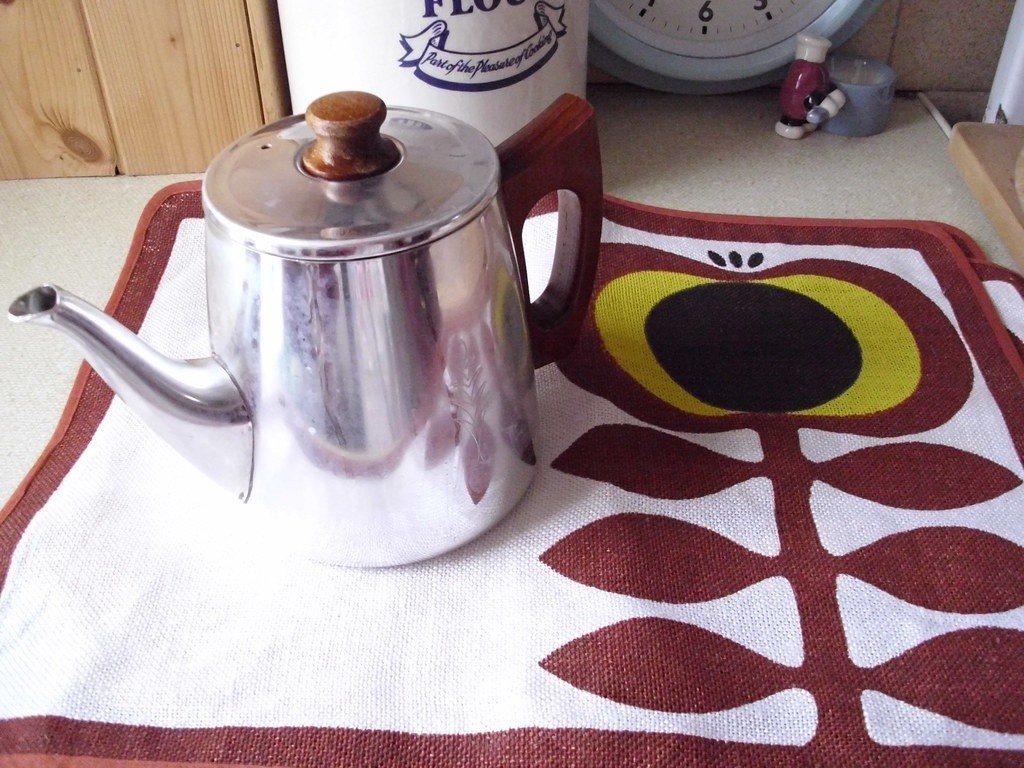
[8,92,600,569]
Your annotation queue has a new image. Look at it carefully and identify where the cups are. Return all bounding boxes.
[376,1,588,142]
